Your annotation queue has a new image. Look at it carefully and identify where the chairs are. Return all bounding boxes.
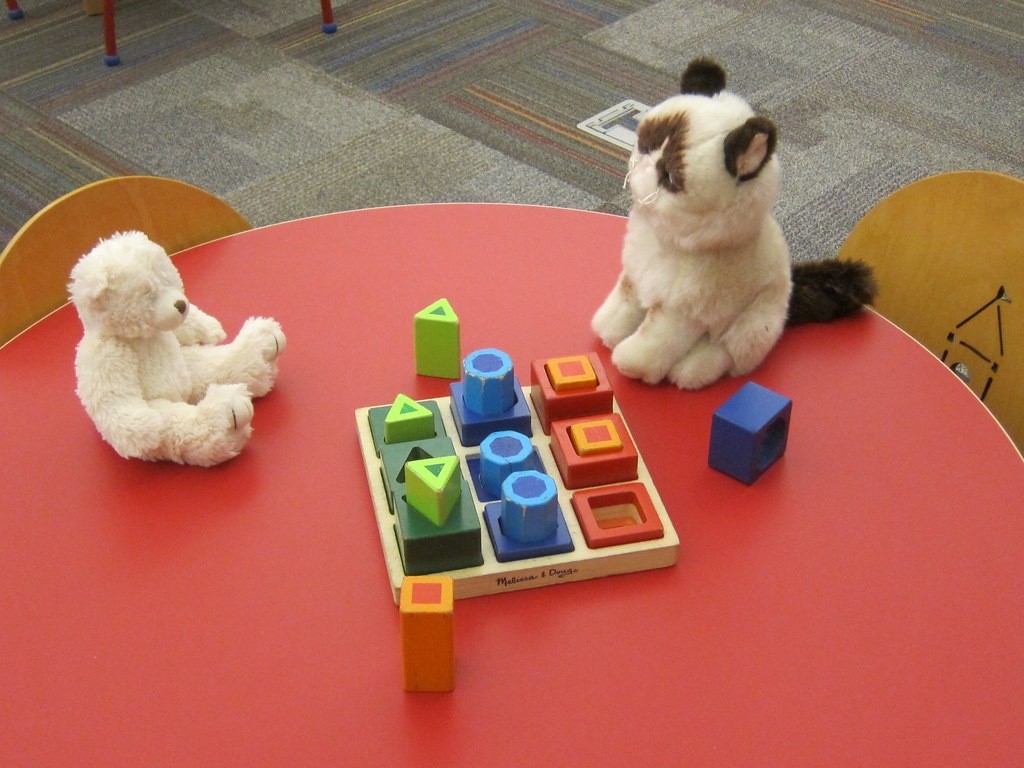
[1,176,254,348]
[834,171,1024,455]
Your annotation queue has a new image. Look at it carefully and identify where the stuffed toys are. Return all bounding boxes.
[67,231,286,465]
[592,58,876,390]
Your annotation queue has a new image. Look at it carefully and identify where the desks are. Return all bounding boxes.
[0,202,1024,768]
